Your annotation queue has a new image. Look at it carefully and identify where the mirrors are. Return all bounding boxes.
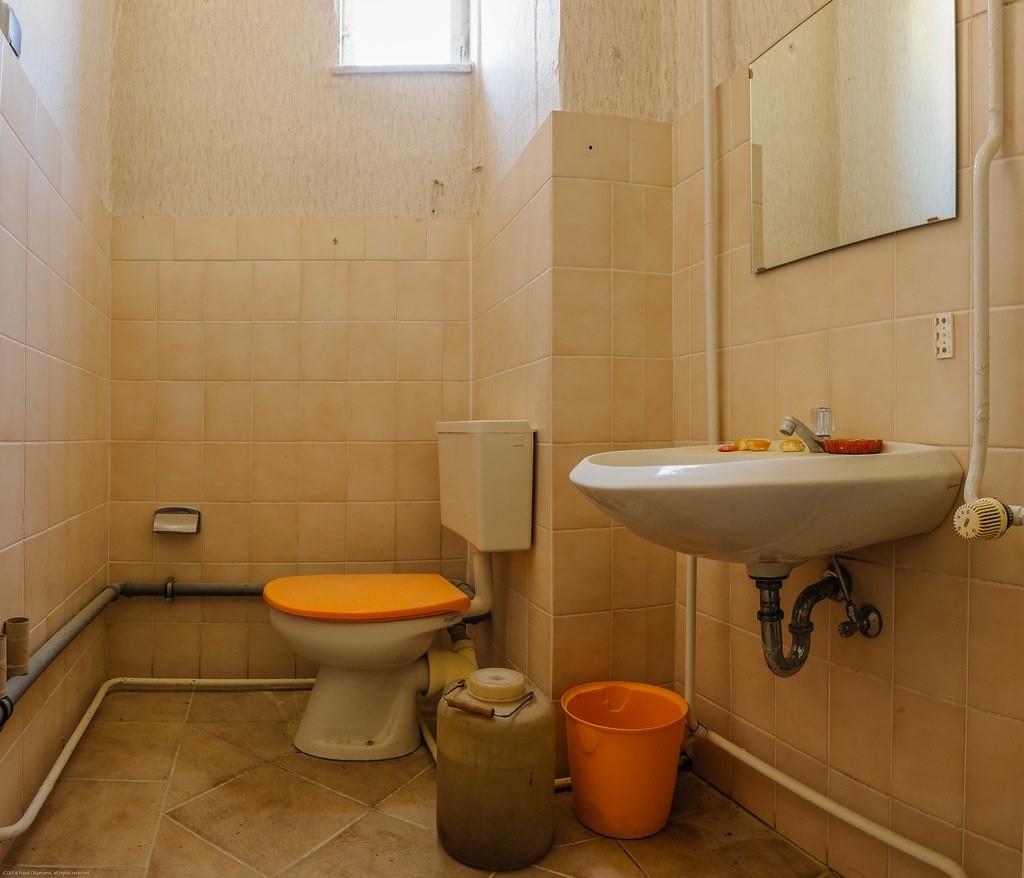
[749,0,959,274]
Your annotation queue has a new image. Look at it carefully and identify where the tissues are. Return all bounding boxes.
[151,506,203,536]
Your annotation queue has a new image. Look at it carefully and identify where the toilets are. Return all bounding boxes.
[260,417,539,762]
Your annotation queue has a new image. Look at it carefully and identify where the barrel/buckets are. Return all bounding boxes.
[433,668,556,868]
[562,681,691,840]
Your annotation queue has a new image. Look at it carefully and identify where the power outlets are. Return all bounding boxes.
[934,312,955,360]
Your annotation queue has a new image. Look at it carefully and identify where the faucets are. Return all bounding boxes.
[779,405,833,453]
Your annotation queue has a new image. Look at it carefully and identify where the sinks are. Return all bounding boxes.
[569,445,964,579]
